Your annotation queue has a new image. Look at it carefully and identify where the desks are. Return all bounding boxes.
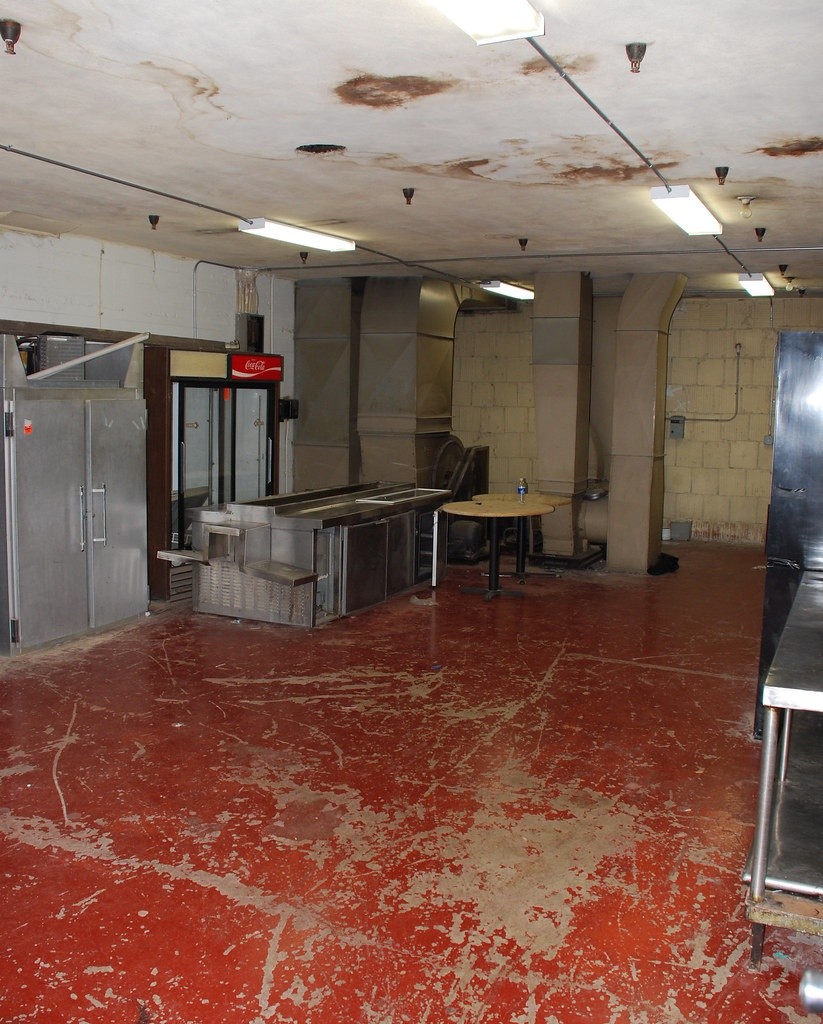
[472,493,572,585]
[743,569,823,968]
[442,500,554,602]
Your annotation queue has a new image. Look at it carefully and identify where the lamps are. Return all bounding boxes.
[651,186,723,237]
[479,280,534,300]
[434,0,546,46]
[738,274,774,297]
[239,218,356,252]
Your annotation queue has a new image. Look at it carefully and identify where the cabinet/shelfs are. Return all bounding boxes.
[272,510,416,614]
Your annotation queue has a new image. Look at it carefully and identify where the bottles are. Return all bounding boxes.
[518,478,527,504]
